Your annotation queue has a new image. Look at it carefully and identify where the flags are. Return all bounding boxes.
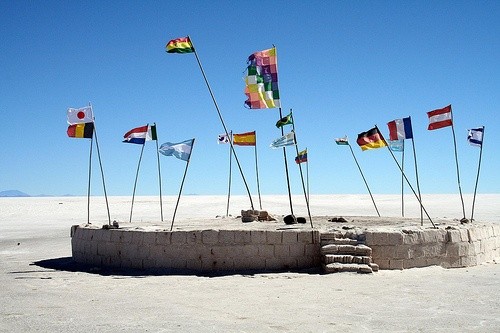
[217,132,233,144]
[66,105,94,126]
[466,127,484,148]
[335,137,350,146]
[159,138,193,161]
[233,131,257,147]
[242,47,282,110]
[294,149,308,164]
[270,132,298,149]
[275,112,294,129]
[66,117,95,139]
[356,126,389,151]
[165,36,196,54]
[385,138,405,152]
[426,105,454,131]
[122,125,149,144]
[145,124,158,141]
[386,116,414,141]
